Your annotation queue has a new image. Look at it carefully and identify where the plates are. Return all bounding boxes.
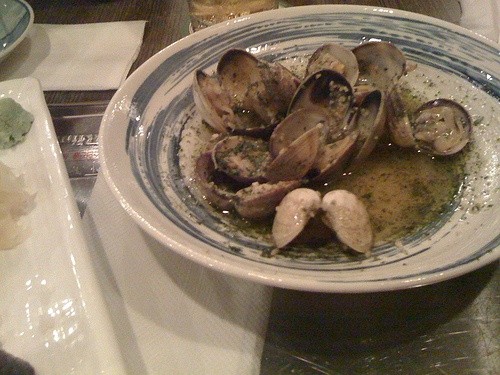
[0,77,128,375]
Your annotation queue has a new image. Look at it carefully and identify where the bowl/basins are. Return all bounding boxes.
[101,4,500,292]
[0,0,34,63]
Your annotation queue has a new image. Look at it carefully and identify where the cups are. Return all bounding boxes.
[188,0,279,33]
